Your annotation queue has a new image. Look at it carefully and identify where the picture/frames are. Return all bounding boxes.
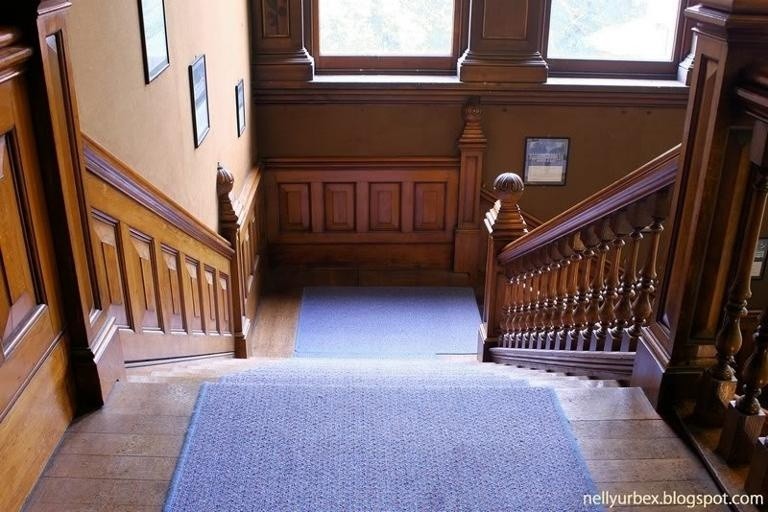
[233,77,248,140]
[136,0,169,85]
[522,135,571,188]
[186,53,211,151]
